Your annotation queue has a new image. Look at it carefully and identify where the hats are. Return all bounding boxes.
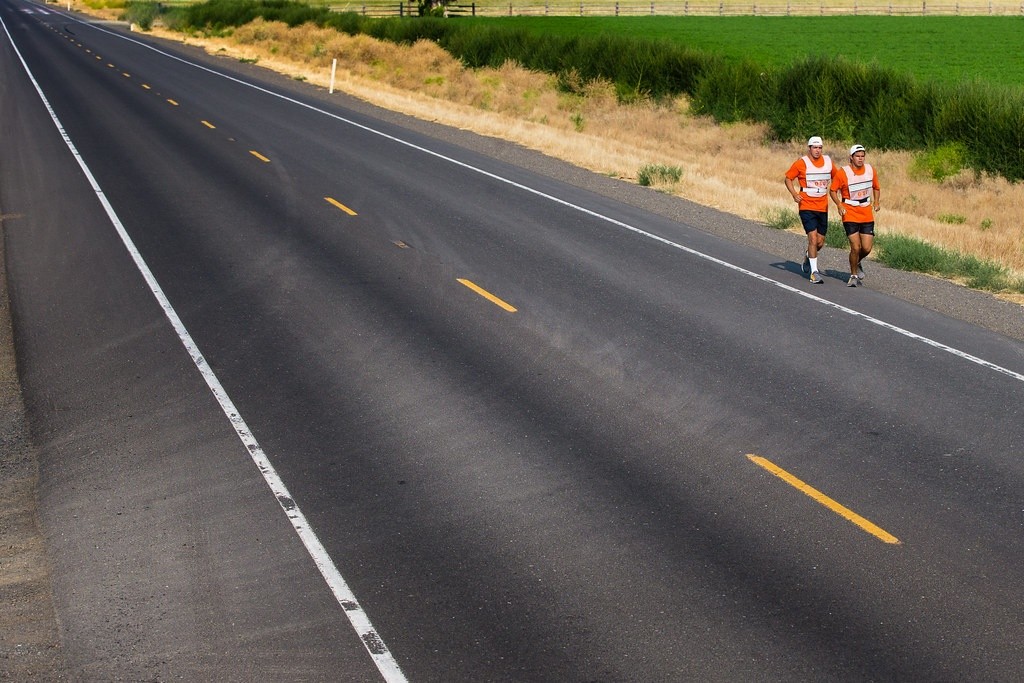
[849,145,865,163]
[807,137,823,147]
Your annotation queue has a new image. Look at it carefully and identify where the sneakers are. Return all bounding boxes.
[856,263,865,279]
[847,276,858,287]
[802,249,811,273]
[809,271,824,284]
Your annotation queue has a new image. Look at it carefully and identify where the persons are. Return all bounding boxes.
[784,136,839,284]
[829,144,881,288]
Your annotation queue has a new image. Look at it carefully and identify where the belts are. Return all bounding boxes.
[842,196,871,206]
[800,187,828,193]
